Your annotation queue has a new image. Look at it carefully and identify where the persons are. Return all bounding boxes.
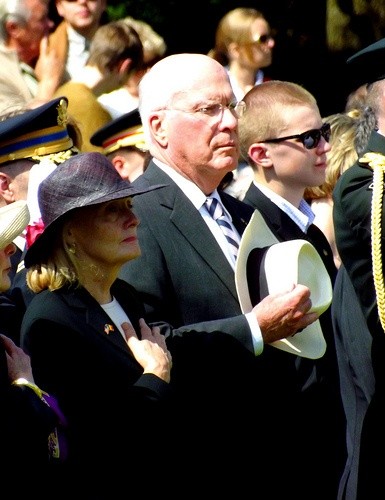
[114,53,318,500]
[206,0,385,500]
[0,0,166,289]
[20,153,172,500]
[0,203,70,500]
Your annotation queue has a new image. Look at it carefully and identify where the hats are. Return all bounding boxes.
[235,209,333,361]
[346,39,385,84]
[0,97,79,166]
[0,200,31,249]
[24,153,168,268]
[89,107,148,153]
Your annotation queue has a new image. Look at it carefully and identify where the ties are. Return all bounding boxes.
[204,198,240,260]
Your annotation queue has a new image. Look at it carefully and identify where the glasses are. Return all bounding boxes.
[252,33,273,45]
[157,100,246,120]
[260,123,331,149]
